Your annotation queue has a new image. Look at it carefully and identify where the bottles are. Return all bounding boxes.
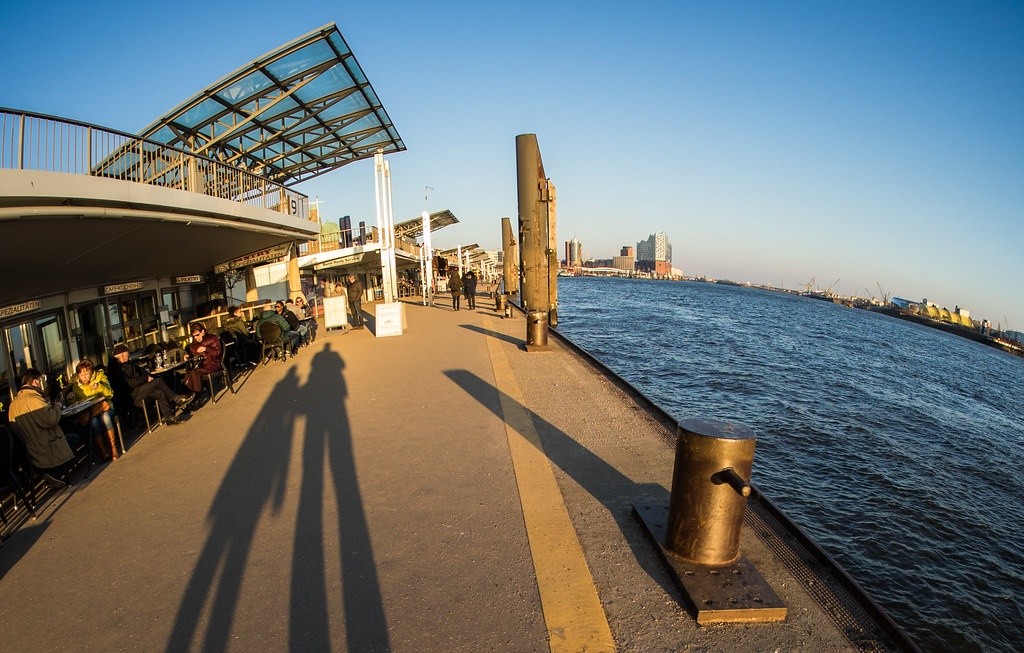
[155,350,170,371]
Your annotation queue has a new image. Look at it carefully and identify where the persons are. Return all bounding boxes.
[347,274,364,329]
[331,282,347,307]
[405,255,505,310]
[8,297,313,489]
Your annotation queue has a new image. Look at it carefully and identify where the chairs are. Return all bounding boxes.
[0,321,289,540]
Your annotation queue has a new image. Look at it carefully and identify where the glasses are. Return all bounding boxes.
[275,305,282,308]
[192,332,200,337]
[297,300,302,303]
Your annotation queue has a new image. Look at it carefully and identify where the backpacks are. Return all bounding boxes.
[452,283,458,292]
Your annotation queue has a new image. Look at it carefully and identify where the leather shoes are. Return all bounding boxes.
[167,417,186,426]
[187,392,203,411]
[176,392,195,409]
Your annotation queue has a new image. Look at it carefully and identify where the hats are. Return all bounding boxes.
[113,344,127,355]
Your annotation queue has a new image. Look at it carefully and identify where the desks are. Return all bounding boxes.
[299,317,313,345]
[59,396,106,469]
[149,358,187,393]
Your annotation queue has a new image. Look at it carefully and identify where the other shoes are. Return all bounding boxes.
[285,350,294,359]
[267,351,271,357]
[350,326,358,331]
[42,472,66,489]
[277,351,282,363]
[357,326,363,330]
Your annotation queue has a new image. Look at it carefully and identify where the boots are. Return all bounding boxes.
[103,428,120,460]
[94,434,111,462]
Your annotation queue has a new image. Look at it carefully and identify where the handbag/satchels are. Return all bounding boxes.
[78,401,111,427]
[185,353,203,372]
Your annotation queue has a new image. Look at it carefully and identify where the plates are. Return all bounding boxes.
[59,396,99,415]
